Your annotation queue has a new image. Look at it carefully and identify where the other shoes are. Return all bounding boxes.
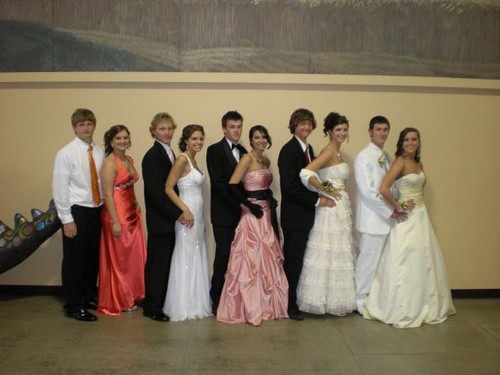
[289,313,304,321]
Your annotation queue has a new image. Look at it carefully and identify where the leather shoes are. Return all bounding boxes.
[86,300,97,310]
[147,312,170,322]
[68,307,98,322]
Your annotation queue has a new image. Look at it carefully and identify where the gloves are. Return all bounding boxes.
[272,198,278,209]
[229,184,263,218]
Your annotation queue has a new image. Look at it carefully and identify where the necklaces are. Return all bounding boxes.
[252,150,265,165]
[327,145,342,159]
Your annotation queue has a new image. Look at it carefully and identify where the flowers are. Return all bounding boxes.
[378,153,387,168]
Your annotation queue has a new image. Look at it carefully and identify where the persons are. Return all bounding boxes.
[363,127,456,328]
[162,124,214,321]
[142,112,185,321]
[52,108,106,320]
[277,108,336,321]
[354,115,408,315]
[216,124,290,326]
[206,110,248,315]
[295,112,356,317]
[96,124,145,315]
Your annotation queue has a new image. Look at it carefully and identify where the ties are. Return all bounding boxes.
[304,149,309,164]
[88,145,101,206]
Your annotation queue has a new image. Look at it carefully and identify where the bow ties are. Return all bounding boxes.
[232,143,241,150]
